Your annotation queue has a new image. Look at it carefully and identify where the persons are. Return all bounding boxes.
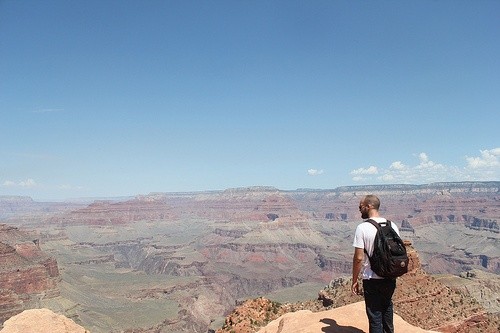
[351,194,401,333]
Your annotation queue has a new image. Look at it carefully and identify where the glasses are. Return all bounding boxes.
[358,204,365,209]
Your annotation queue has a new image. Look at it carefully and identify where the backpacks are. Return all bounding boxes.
[364,219,409,278]
